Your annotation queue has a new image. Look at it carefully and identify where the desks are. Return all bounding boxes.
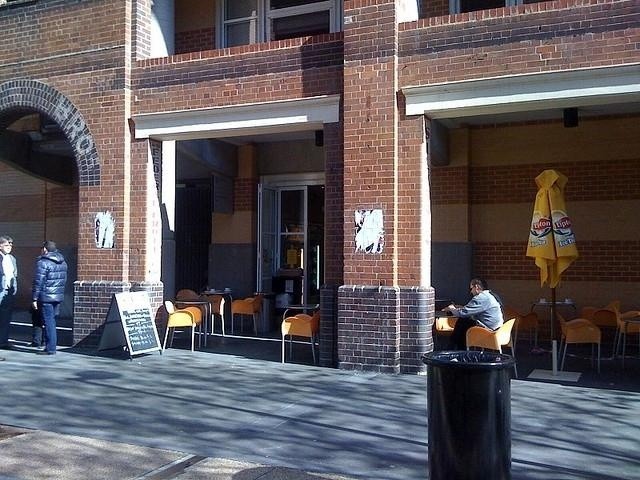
[174,301,209,348]
[283,304,319,345]
[620,316,640,375]
[532,301,576,348]
[200,289,233,304]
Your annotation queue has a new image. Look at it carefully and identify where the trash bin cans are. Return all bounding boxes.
[421,349,515,480]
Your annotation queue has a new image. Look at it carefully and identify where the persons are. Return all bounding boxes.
[26,303,45,348]
[0,234,19,350]
[31,240,68,357]
[444,277,505,351]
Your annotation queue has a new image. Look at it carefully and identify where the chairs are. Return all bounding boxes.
[231,294,263,337]
[280,310,321,365]
[176,289,199,299]
[163,300,202,355]
[504,305,538,356]
[591,308,617,357]
[612,299,640,359]
[435,315,463,351]
[578,305,599,321]
[466,317,519,379]
[207,295,225,339]
[556,310,602,377]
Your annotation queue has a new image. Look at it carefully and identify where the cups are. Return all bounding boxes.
[225,288,230,290]
[540,298,545,301]
[211,289,215,291]
[565,298,571,302]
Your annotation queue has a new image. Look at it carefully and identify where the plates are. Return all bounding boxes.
[565,302,573,304]
[209,291,216,293]
[540,302,548,304]
[549,302,562,304]
[224,290,232,291]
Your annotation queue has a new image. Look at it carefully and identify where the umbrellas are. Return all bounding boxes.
[523,168,579,376]
